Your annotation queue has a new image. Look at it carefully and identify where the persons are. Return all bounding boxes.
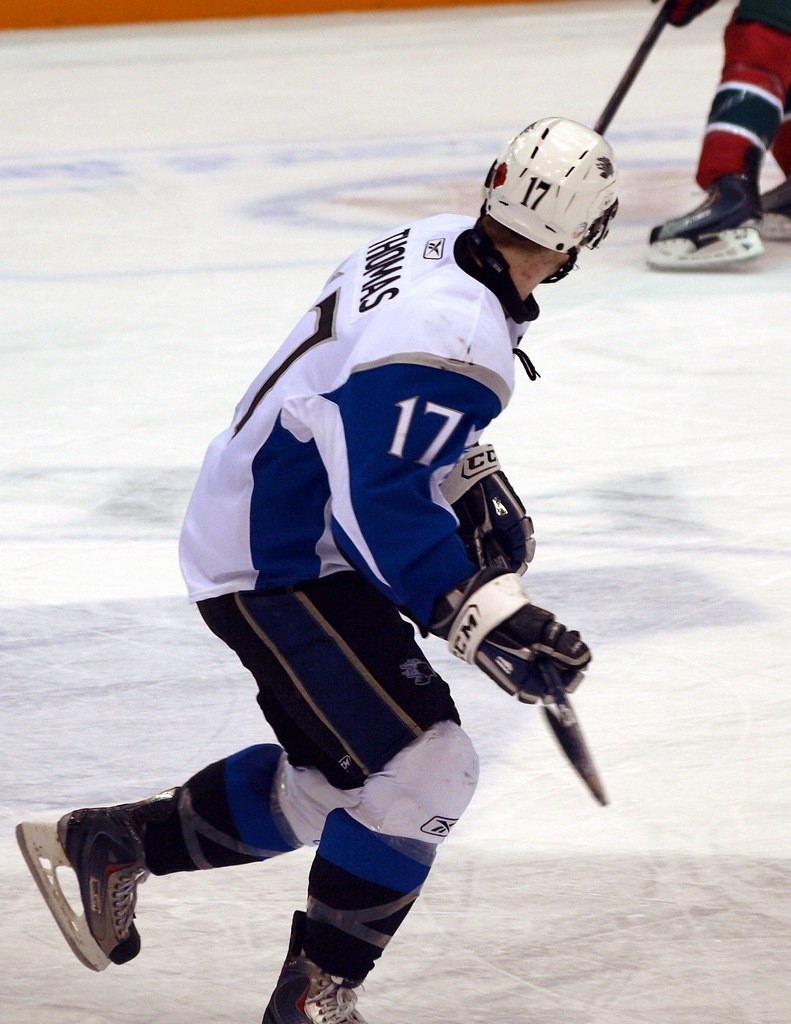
[15,117,618,1024]
[646,0,791,273]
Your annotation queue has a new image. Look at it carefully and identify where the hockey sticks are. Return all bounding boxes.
[475,516,608,808]
[591,6,673,142]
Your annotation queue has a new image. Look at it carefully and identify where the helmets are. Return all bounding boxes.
[482,117,617,254]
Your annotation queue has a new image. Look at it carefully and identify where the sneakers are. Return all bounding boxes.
[15,787,183,972]
[261,910,370,1024]
[756,200,791,240]
[647,145,764,268]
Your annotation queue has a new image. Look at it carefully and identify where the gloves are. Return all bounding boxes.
[440,444,536,578]
[428,567,592,705]
[651,0,719,27]
